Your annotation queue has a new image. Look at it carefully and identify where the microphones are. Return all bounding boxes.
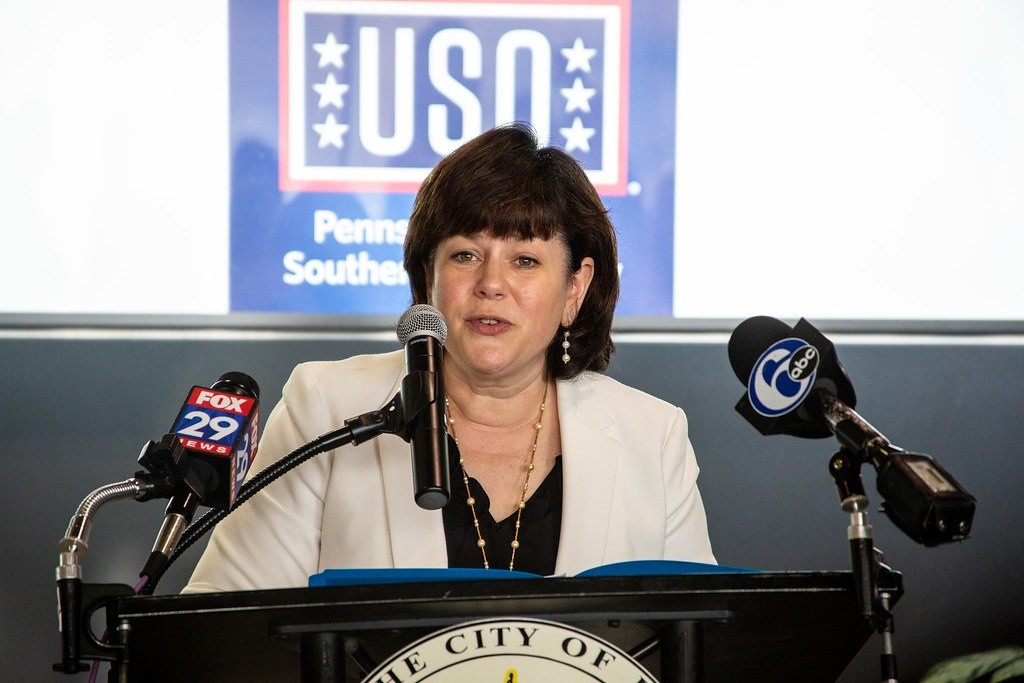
[140,370,261,586]
[727,317,976,548]
[397,305,451,510]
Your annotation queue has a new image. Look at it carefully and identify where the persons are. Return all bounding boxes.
[181,120,717,594]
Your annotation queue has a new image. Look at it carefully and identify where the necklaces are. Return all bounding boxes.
[445,376,549,572]
